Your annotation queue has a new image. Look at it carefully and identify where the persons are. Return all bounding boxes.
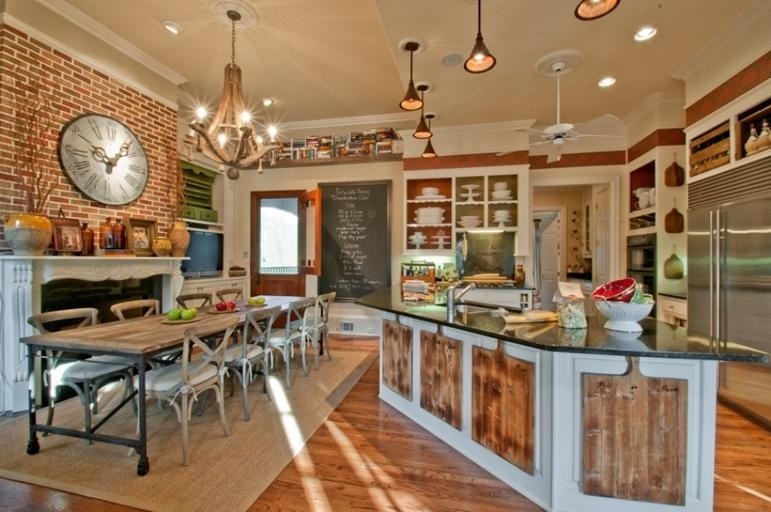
[131,225,149,250]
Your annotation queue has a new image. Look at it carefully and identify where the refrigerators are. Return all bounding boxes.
[687,194,771,429]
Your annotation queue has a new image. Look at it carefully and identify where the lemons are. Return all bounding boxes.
[255,297,266,305]
[248,298,255,305]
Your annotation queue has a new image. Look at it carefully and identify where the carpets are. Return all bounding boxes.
[0,346,380,512]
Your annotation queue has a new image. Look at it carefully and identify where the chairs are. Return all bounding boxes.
[196,305,282,421]
[126,316,240,466]
[282,291,337,370]
[113,300,180,413]
[217,290,261,386]
[251,296,315,388]
[178,292,236,396]
[26,307,135,446]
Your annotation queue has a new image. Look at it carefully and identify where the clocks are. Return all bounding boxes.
[56,113,149,207]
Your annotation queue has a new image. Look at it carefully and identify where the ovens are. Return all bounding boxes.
[626,232,657,317]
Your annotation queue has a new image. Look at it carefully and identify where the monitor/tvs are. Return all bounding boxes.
[181,227,225,280]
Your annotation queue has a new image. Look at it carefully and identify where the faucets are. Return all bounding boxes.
[446,281,475,321]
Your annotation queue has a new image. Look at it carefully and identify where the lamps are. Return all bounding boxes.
[421,112,439,159]
[398,43,424,112]
[182,0,279,180]
[463,0,496,74]
[412,81,433,139]
[574,0,621,22]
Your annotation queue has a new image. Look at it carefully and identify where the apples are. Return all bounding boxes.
[168,310,180,320]
[190,308,197,318]
[181,310,192,320]
[227,301,235,310]
[216,303,226,311]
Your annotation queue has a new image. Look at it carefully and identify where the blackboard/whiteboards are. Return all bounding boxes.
[317,179,392,303]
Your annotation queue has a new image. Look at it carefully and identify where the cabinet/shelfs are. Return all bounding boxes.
[405,167,454,253]
[454,164,530,257]
[616,145,688,324]
[175,274,249,310]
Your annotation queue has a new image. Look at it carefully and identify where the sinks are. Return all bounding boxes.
[456,303,501,315]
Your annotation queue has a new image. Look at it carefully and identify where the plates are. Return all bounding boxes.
[246,303,266,308]
[206,307,240,315]
[457,216,482,228]
[161,316,202,325]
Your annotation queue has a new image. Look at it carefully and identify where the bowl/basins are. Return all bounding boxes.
[639,187,655,209]
[592,278,636,302]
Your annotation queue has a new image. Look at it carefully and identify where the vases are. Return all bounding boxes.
[167,221,190,257]
[3,213,53,256]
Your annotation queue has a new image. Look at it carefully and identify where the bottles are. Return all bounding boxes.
[99,217,115,249]
[78,222,94,256]
[113,217,127,248]
[407,263,447,278]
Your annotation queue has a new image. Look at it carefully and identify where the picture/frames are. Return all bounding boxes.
[123,214,157,252]
[51,216,83,252]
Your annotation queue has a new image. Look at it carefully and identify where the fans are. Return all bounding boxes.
[494,49,627,164]
